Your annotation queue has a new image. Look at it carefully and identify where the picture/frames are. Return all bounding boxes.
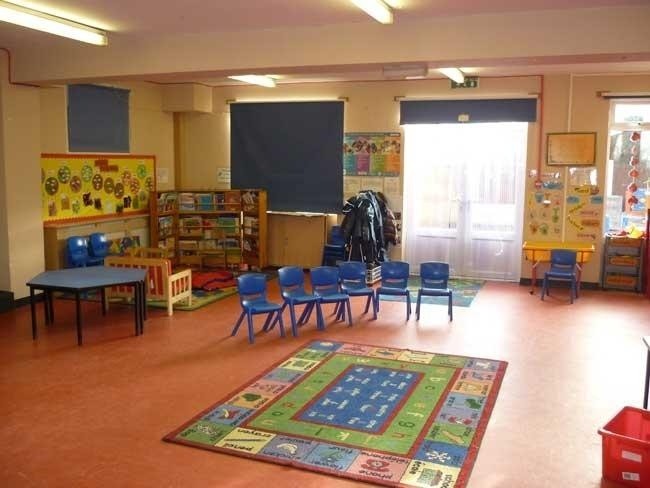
[545,131,597,167]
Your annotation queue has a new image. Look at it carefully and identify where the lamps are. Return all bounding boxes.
[0,0,109,49]
[349,0,407,24]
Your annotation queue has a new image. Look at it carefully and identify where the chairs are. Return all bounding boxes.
[231,273,288,343]
[415,262,455,321]
[270,267,323,332]
[66,236,103,300]
[87,233,109,262]
[375,260,412,320]
[543,249,578,303]
[305,265,352,329]
[324,225,346,265]
[337,262,379,324]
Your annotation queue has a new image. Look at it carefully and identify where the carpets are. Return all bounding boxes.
[162,337,508,487]
[56,272,274,312]
[379,274,485,306]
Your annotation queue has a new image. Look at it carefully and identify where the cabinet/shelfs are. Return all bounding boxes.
[268,209,338,271]
[150,187,177,271]
[176,188,268,271]
[600,233,647,291]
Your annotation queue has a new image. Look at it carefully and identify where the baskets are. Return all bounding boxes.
[338,266,382,284]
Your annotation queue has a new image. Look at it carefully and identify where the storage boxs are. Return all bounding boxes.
[598,403,650,487]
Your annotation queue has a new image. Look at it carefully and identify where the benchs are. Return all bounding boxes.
[104,255,193,317]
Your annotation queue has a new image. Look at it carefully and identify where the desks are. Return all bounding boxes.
[25,266,149,346]
[523,240,595,294]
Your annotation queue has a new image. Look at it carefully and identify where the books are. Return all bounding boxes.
[155,191,259,271]
